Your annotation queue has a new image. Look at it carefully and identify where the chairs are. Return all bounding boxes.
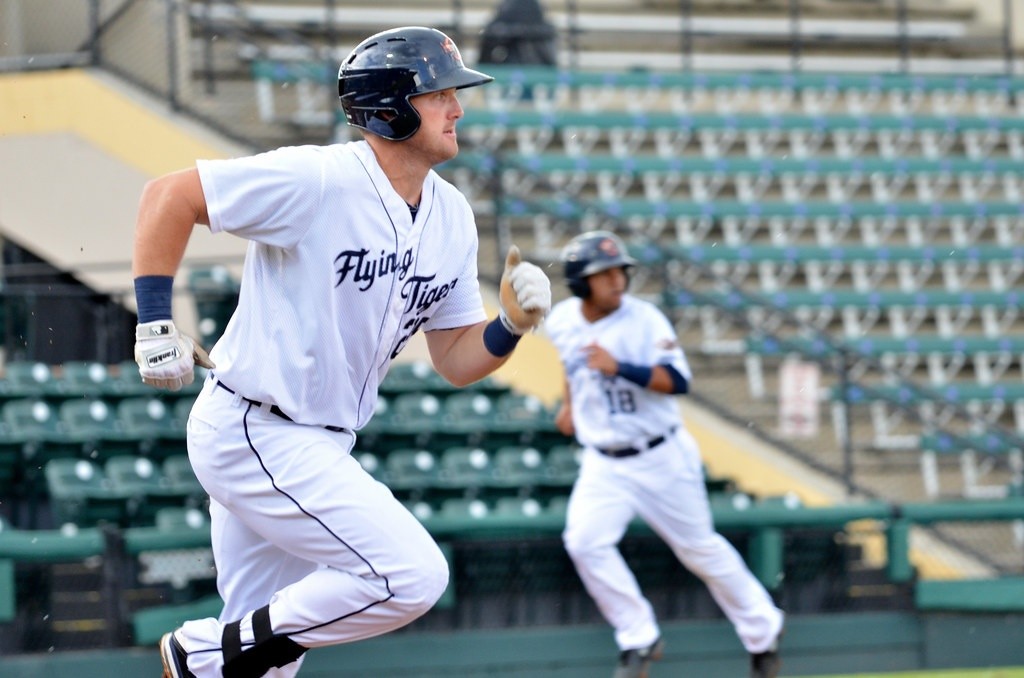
[2,66,1021,629]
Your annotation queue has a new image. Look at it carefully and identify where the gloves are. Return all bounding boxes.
[134,319,214,391]
[498,244,552,332]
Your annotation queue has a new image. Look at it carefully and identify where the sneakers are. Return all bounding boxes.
[158,630,199,678]
[746,627,785,678]
[612,637,665,678]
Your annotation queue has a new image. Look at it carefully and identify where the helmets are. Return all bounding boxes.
[559,230,639,298]
[338,26,495,143]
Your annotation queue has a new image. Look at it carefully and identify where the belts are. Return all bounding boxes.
[206,369,346,434]
[589,423,681,458]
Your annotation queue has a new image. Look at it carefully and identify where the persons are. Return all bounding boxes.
[538,230,788,676]
[129,27,551,670]
